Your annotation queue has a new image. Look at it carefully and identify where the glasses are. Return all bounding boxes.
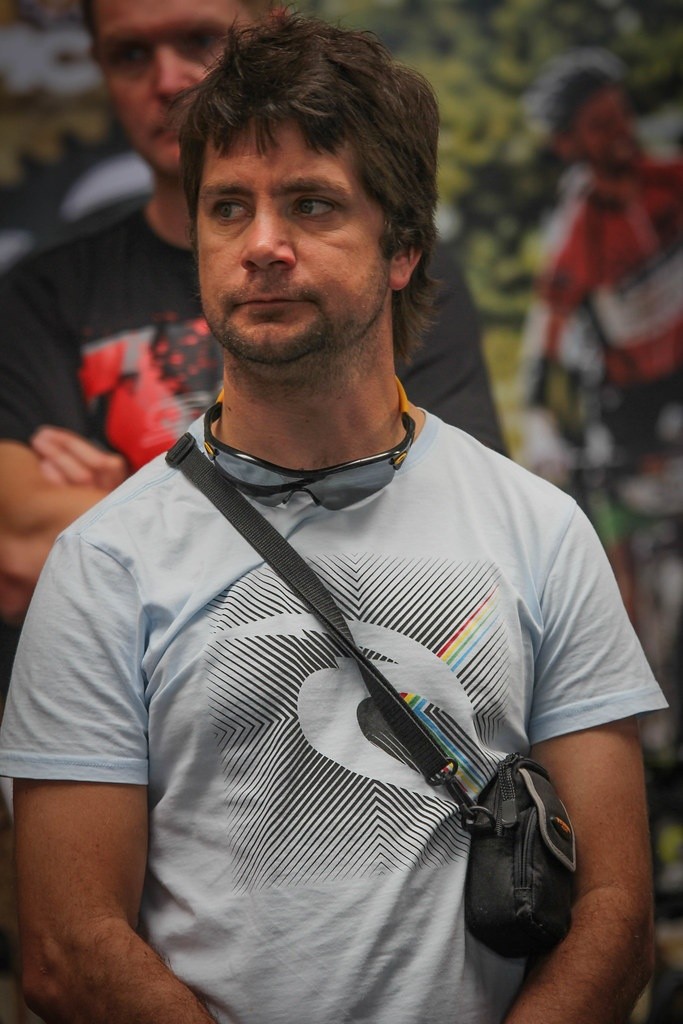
[204,402,416,510]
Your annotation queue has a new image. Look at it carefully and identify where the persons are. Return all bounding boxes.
[0,0,683,1024]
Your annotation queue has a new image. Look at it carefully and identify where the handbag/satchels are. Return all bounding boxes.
[465,753,573,955]
